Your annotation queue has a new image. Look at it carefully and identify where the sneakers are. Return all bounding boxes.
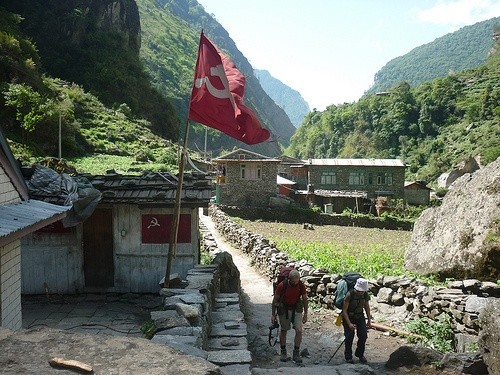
[355,355,367,362]
[346,359,353,363]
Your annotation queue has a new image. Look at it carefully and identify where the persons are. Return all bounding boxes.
[270,269,308,363]
[341,278,371,363]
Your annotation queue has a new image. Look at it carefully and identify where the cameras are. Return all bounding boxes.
[269,320,279,331]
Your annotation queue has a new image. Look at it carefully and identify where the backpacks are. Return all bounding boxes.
[273,267,302,310]
[332,272,367,310]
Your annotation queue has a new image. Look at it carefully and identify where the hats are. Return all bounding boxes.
[289,270,300,278]
[354,278,368,291]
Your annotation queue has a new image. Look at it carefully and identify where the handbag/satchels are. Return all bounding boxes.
[277,303,284,315]
[296,300,304,313]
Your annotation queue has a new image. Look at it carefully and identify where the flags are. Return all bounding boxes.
[187,28,272,145]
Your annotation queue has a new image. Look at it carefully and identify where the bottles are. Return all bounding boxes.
[335,314,342,326]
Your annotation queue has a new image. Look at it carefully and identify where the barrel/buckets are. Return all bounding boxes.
[325,204,332,213]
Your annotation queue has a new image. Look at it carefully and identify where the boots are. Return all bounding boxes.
[293,349,302,363]
[280,349,287,362]
